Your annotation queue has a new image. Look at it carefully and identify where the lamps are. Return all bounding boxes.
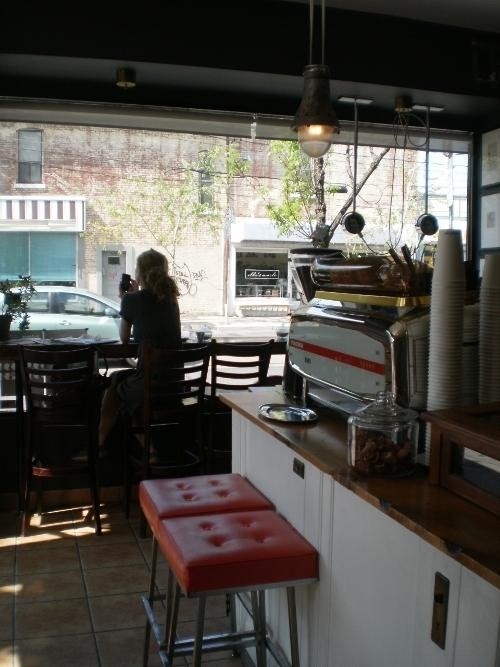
[292,0,338,155]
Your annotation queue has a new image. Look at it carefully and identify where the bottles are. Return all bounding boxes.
[347,391,420,477]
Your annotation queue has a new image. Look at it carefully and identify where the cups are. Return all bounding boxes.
[200,326,212,342]
[426,228,500,467]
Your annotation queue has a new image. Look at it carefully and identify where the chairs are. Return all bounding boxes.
[124,342,212,519]
[17,345,103,534]
[208,339,276,472]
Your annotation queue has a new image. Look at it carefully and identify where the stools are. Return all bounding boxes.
[139,474,318,667]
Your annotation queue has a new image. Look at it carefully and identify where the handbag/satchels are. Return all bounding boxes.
[46,369,112,408]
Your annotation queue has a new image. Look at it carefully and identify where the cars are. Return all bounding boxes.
[0,284,121,340]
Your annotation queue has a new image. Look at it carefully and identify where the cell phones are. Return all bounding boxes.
[119,273,131,291]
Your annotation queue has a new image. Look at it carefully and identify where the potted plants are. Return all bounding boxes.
[0,275,35,341]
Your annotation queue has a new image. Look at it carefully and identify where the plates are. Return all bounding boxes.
[259,403,319,424]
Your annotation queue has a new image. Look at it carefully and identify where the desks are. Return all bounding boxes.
[2,339,287,493]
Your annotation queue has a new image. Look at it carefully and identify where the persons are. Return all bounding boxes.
[71,247,184,462]
